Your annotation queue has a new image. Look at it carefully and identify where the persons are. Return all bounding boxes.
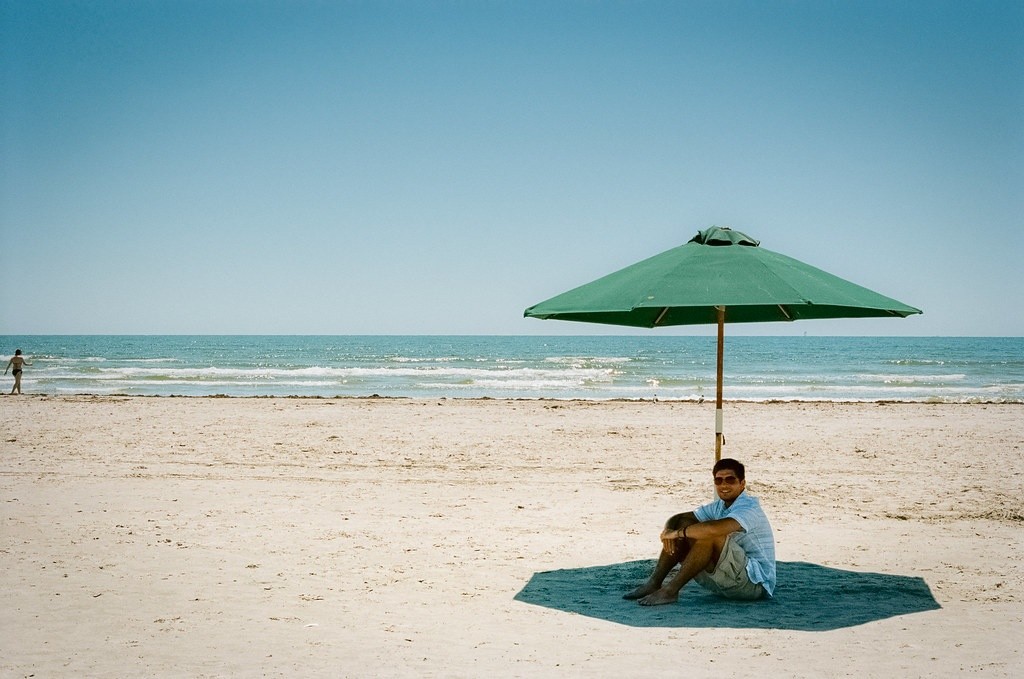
[622,458,777,607]
[4,348,33,394]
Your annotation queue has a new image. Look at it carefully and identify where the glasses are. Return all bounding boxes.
[714,476,741,486]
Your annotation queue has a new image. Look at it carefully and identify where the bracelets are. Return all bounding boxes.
[677,527,684,541]
[683,525,690,537]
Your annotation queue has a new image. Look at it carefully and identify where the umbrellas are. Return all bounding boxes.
[524,227,924,501]
